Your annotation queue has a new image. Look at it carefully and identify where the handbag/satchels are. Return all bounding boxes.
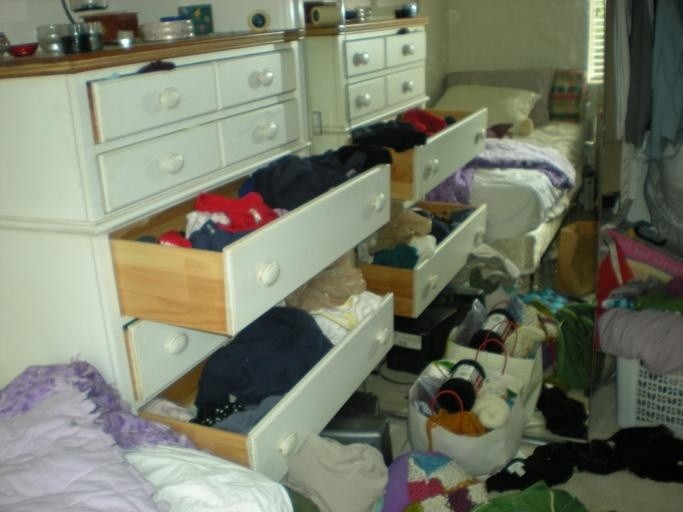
[409,326,544,480]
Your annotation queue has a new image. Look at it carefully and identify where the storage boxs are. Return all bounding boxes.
[553,220,605,299]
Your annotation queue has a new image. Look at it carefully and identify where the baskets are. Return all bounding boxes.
[616,357,683,436]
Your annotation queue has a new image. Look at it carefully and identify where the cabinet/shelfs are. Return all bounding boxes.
[1,24,489,483]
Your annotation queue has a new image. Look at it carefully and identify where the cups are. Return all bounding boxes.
[67,22,103,55]
[35,23,70,57]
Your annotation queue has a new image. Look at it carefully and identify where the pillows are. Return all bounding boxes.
[439,67,588,131]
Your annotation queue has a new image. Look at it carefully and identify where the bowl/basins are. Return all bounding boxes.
[4,42,39,58]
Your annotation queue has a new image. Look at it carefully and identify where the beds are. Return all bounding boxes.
[416,119,586,283]
